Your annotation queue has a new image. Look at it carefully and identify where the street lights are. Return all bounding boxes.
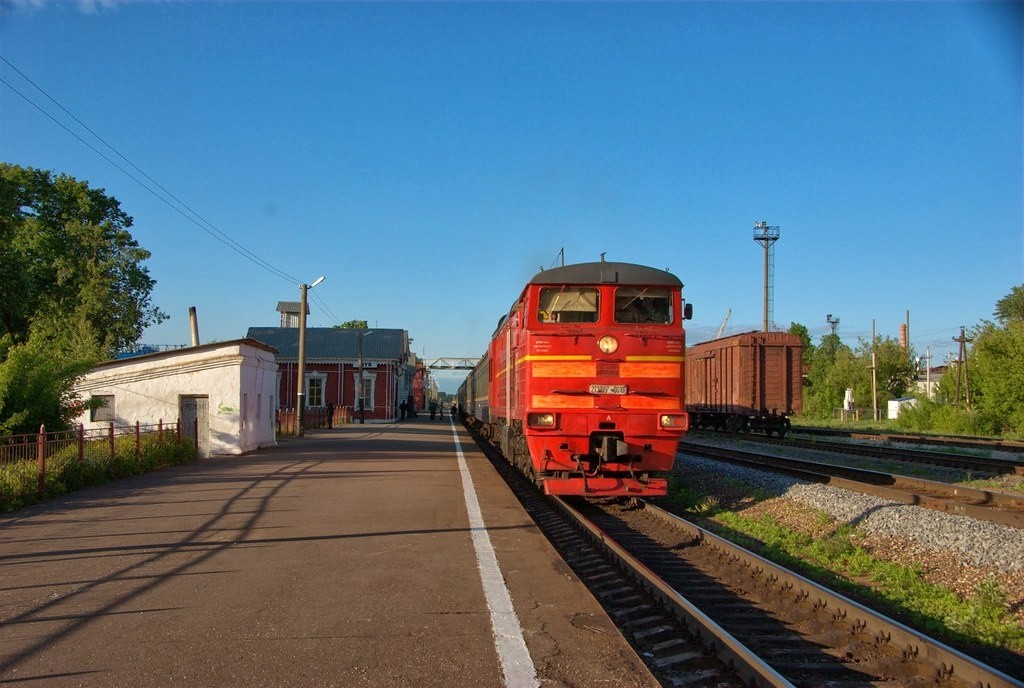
[356,330,374,424]
[294,275,325,435]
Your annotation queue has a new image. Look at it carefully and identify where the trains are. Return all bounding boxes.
[683,330,804,438]
[454,245,694,505]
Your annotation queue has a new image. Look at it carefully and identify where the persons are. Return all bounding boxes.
[399,400,406,422]
[439,402,444,420]
[325,400,335,429]
[451,404,457,421]
